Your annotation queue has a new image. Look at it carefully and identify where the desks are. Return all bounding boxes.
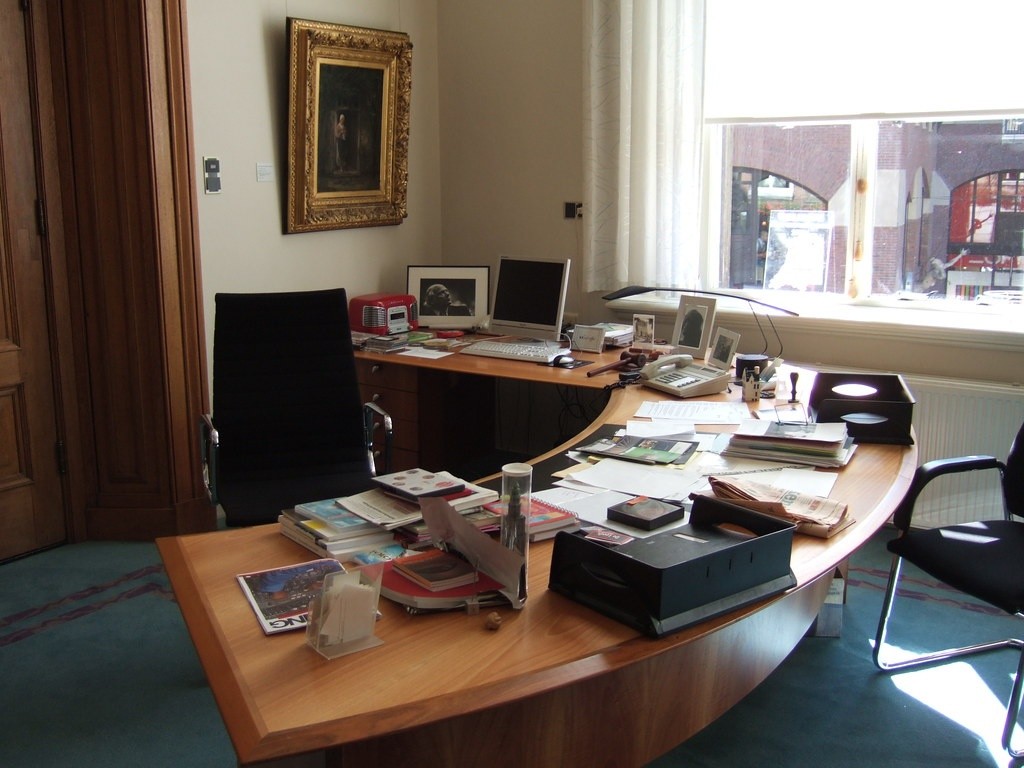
[155,328,918,768]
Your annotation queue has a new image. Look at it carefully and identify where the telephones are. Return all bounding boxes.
[639,354,732,398]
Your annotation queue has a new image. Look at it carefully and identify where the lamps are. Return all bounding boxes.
[602,285,799,358]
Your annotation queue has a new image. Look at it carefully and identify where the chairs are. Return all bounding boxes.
[200,288,392,528]
[872,420,1024,759]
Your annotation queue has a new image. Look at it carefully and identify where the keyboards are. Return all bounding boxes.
[459,341,571,363]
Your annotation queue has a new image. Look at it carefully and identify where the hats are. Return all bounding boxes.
[423,283,448,309]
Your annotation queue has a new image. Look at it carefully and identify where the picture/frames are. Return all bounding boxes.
[284,17,413,235]
[570,324,606,353]
[708,327,740,371]
[407,265,490,328]
[672,295,716,359]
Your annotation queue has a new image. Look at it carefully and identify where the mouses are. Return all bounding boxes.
[552,355,575,365]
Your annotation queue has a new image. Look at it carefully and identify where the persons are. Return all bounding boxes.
[748,218,774,286]
[420,284,472,316]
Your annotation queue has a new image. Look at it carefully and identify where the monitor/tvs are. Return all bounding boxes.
[489,255,570,341]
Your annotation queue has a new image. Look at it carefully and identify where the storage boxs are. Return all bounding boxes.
[607,496,685,532]
[814,578,844,637]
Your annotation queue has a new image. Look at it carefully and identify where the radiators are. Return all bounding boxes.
[784,362,1024,531]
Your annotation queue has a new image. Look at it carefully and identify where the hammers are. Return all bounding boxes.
[587,351,647,378]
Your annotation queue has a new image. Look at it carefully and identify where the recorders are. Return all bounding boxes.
[347,294,418,337]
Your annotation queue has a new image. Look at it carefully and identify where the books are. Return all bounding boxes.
[576,434,700,464]
[237,469,581,642]
[723,419,858,468]
